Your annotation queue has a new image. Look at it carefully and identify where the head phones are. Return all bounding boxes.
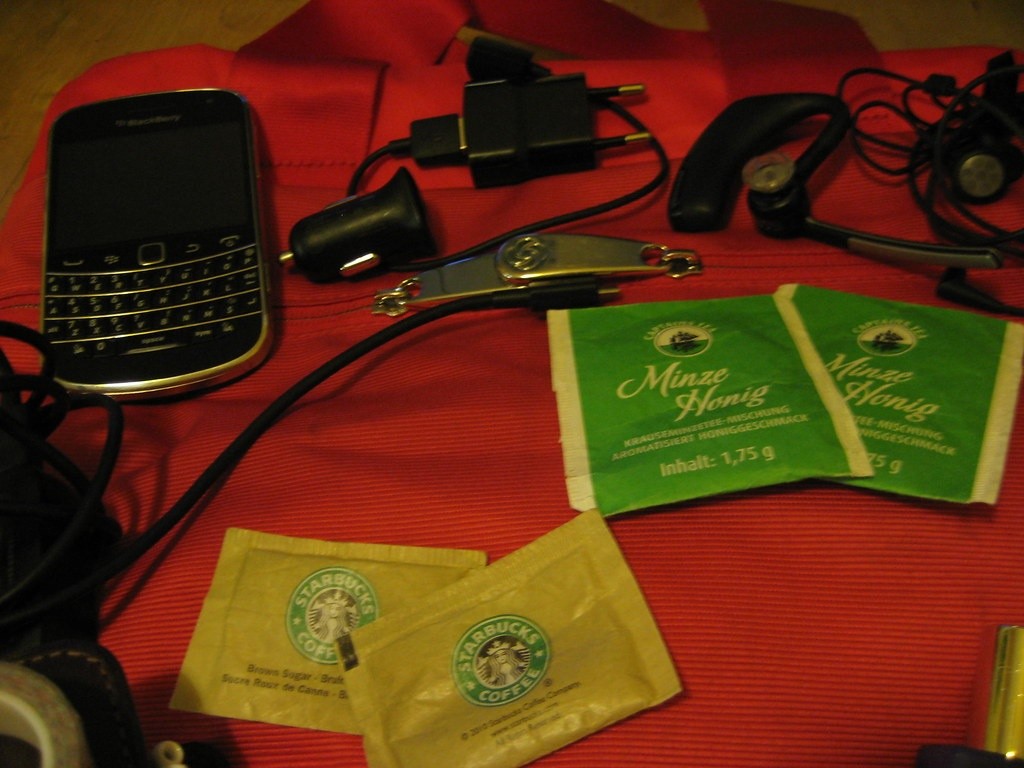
[936,92,1024,202]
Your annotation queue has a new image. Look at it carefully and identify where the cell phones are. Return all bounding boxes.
[44,88,273,401]
